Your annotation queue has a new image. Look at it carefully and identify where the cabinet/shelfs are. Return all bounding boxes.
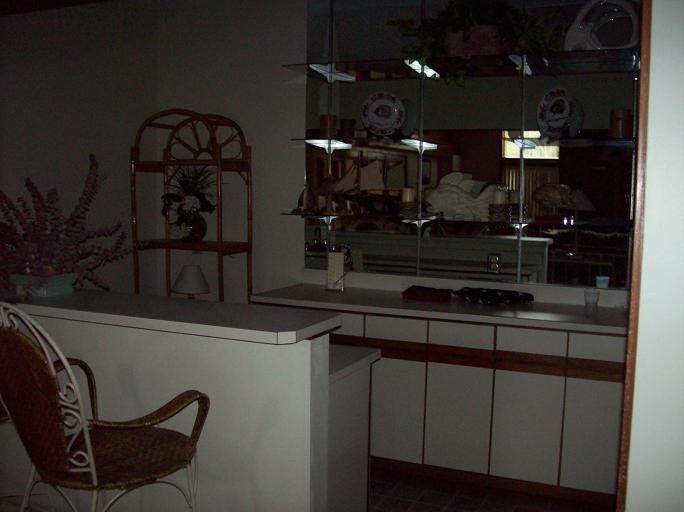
[278,45,639,226]
[129,108,254,304]
[250,284,628,507]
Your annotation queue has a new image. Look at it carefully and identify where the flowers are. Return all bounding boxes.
[0,153,149,303]
[154,155,229,241]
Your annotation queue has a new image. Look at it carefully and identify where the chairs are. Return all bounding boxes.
[0,304,210,512]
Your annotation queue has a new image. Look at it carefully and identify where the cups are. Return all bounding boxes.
[582,288,599,312]
[595,276,610,288]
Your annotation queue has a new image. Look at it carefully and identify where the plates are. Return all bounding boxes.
[538,87,577,139]
[362,91,406,136]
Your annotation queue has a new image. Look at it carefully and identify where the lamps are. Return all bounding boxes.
[170,265,210,300]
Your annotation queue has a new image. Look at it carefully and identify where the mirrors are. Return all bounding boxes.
[304,1,645,290]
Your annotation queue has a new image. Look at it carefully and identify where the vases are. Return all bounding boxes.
[182,212,208,240]
[9,272,79,298]
